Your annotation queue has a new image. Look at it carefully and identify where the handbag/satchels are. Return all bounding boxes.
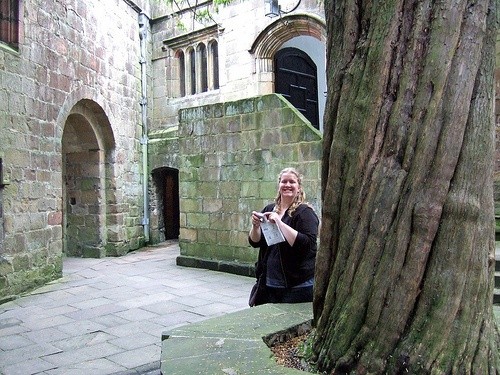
[249,284,259,307]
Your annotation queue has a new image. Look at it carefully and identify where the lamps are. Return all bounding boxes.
[264,1,279,19]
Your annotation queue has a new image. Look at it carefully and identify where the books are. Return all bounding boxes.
[260,220,285,246]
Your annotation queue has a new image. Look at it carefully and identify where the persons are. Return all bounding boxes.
[248,167,319,307]
